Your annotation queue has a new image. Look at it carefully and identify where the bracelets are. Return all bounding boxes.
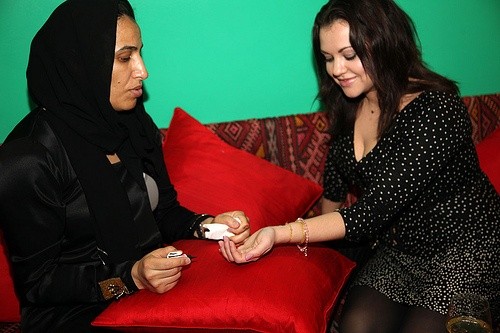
[295,218,309,256]
[285,222,293,244]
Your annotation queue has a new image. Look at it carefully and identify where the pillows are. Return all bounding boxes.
[0,242,21,322]
[90,240,356,333]
[475,125,500,193]
[162,108,325,236]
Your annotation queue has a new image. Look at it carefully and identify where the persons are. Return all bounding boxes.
[218,0,500,333]
[0,0,250,333]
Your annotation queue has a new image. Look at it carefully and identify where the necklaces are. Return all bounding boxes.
[368,100,379,113]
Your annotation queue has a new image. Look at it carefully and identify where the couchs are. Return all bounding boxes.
[0,92,500,333]
